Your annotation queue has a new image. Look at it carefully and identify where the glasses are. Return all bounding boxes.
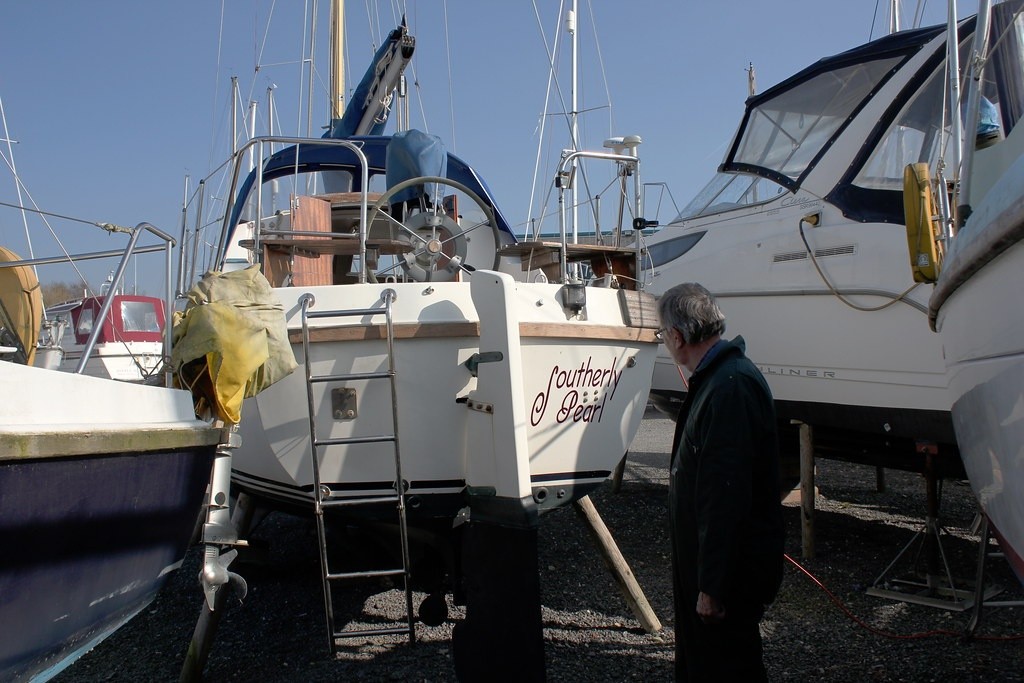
[654,325,670,340]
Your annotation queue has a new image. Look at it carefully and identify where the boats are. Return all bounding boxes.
[899,0,1024,586]
[1,202,220,682]
[59,297,169,384]
[635,0,1006,484]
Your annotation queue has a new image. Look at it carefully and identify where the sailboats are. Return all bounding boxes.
[159,0,662,577]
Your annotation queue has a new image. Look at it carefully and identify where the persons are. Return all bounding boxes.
[653,282,782,683]
